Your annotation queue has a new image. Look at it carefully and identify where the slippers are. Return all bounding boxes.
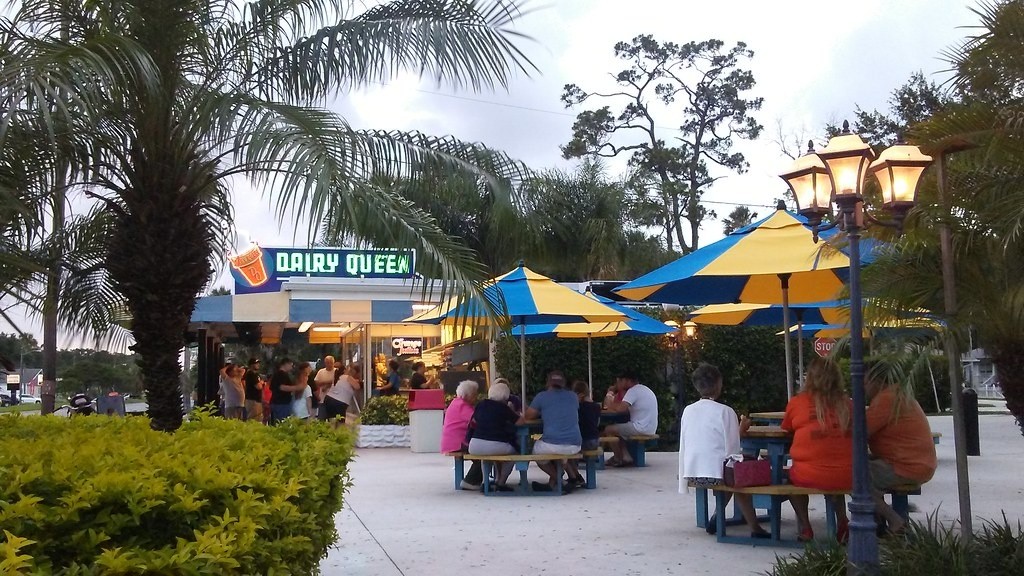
[605,456,633,468]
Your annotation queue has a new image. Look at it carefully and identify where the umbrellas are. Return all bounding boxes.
[402,201,955,405]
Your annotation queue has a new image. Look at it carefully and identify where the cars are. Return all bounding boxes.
[15,394,41,405]
[0,394,18,407]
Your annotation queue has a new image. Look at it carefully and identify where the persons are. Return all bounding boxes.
[524,370,584,493]
[615,371,658,466]
[605,374,628,465]
[781,357,854,545]
[411,362,436,389]
[375,359,400,395]
[218,356,361,422]
[863,362,937,537]
[439,380,483,489]
[468,383,524,491]
[678,365,773,538]
[566,380,601,490]
[493,378,522,417]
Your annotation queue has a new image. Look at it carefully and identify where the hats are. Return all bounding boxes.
[249,358,260,364]
[548,370,565,387]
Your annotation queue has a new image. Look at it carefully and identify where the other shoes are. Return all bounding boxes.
[837,517,850,546]
[750,530,770,540]
[460,480,483,490]
[798,528,813,540]
[706,515,717,535]
[490,482,514,491]
[532,474,552,492]
[881,519,907,539]
[564,474,585,488]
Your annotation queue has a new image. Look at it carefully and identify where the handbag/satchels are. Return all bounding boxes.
[723,456,771,487]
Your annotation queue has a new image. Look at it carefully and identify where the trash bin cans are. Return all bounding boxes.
[407,389,446,453]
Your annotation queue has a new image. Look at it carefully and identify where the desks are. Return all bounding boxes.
[601,410,629,415]
[740,412,837,540]
[515,419,543,488]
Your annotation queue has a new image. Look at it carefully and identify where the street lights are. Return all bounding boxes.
[781,134,934,572]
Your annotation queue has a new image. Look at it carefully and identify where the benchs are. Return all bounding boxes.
[445,434,659,496]
[688,482,921,548]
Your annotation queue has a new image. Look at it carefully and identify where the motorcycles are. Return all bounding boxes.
[50,393,96,416]
